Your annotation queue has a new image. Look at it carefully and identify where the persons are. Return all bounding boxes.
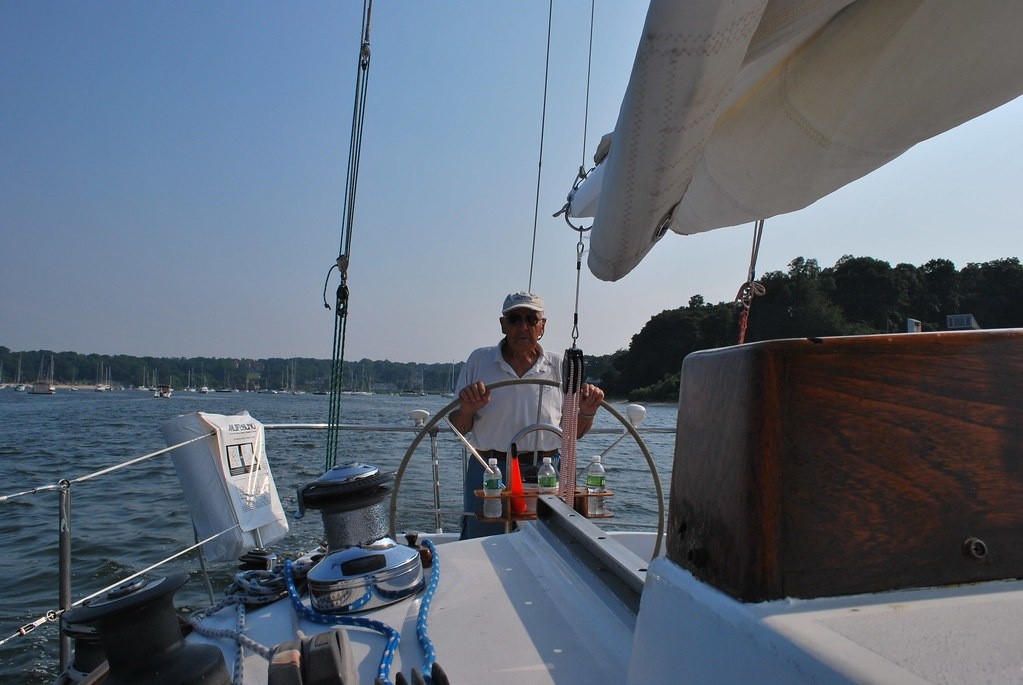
[447,290,605,541]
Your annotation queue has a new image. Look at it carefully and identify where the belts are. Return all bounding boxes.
[475,447,558,465]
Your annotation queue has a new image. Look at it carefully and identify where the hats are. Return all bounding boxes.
[502,290,543,313]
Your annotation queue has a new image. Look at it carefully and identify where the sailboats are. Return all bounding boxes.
[69,364,79,392]
[94,360,125,393]
[126,362,458,400]
[1,350,56,395]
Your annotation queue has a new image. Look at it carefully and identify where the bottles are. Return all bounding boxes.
[587,455,606,515]
[537,457,556,495]
[483,458,502,518]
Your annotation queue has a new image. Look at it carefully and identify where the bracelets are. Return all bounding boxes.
[579,410,596,418]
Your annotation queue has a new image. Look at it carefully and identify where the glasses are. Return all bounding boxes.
[505,314,544,327]
